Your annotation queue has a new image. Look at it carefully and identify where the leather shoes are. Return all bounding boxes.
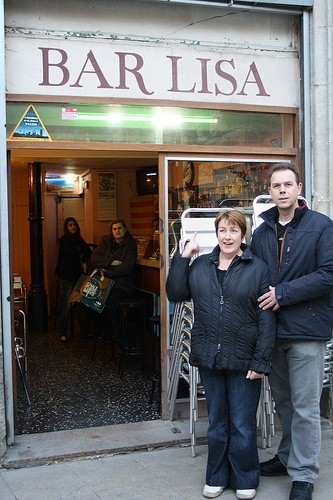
[289,481,314,500]
[259,454,289,477]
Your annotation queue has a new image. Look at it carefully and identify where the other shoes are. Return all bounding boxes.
[236,489,257,499]
[119,337,128,353]
[96,333,110,342]
[202,484,225,498]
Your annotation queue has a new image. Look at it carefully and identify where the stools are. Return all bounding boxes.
[116,300,146,380]
[56,279,115,360]
[150,314,173,410]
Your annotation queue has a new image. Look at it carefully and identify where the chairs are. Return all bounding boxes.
[14,307,31,405]
[170,194,333,458]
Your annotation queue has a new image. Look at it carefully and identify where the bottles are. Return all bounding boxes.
[213,174,250,200]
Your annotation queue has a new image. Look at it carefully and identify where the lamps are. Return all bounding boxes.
[76,112,218,125]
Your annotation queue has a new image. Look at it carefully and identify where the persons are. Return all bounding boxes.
[250,164,333,500]
[91,220,137,343]
[164,210,276,500]
[54,218,91,343]
[144,216,159,260]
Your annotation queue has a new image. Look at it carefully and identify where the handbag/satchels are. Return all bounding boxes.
[67,267,115,313]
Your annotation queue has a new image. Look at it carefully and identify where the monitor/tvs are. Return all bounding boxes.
[136,166,159,196]
